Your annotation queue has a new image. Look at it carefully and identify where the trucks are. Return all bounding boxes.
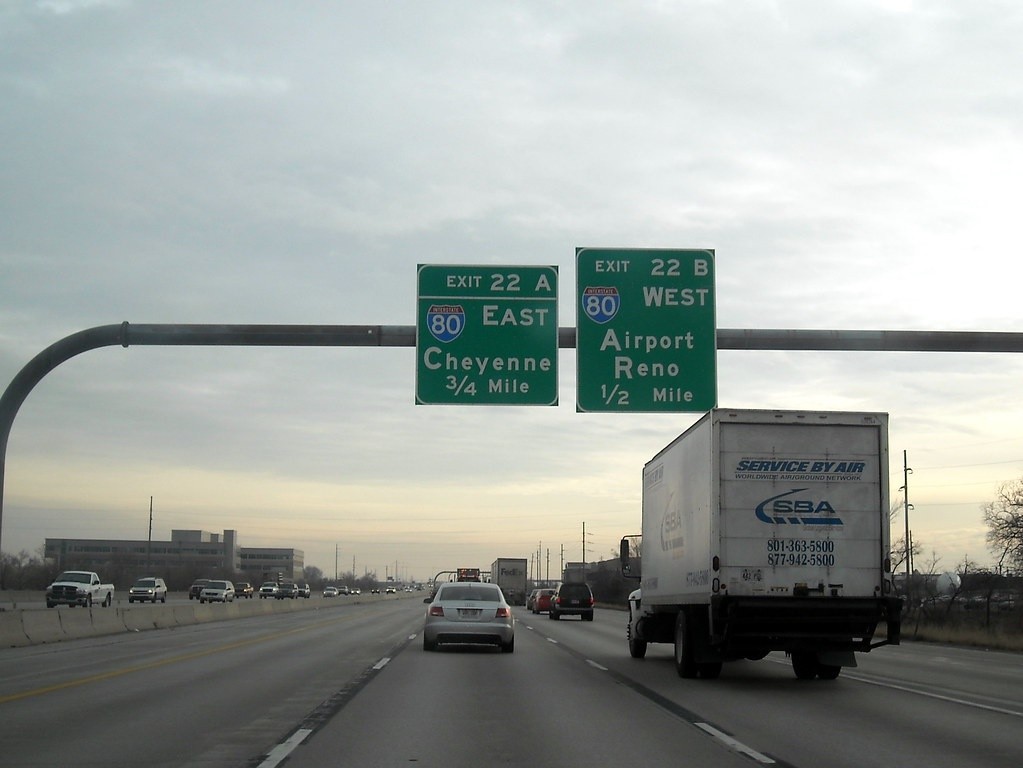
[618,406,894,680]
[490,557,527,606]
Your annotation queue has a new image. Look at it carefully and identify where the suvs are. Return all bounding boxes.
[548,580,594,622]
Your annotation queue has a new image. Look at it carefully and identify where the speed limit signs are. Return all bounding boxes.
[583,285,621,323]
[427,304,466,344]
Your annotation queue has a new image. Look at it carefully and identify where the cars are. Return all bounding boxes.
[531,590,557,614]
[299,584,311,599]
[323,586,339,596]
[526,589,539,610]
[338,585,349,596]
[422,582,515,654]
[189,579,210,600]
[275,583,299,600]
[128,577,167,603]
[235,583,254,599]
[350,588,362,595]
[200,580,236,604]
[371,583,434,595]
[259,582,279,599]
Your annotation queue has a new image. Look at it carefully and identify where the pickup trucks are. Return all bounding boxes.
[46,571,115,608]
